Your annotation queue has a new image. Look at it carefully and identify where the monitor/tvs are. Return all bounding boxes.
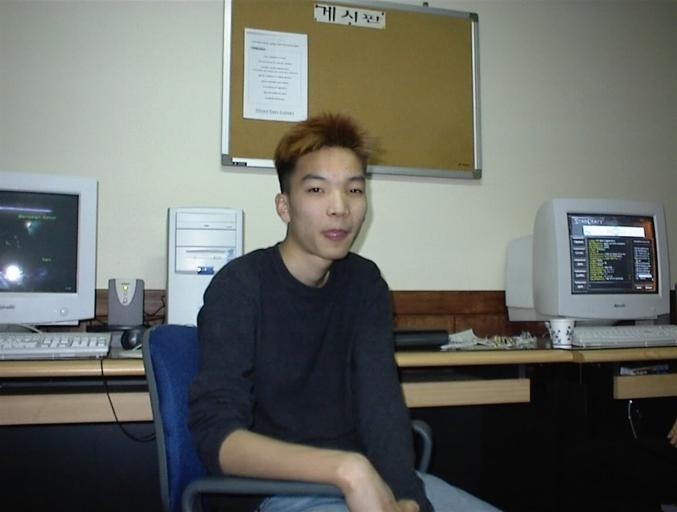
[0,172,97,327]
[504,197,670,321]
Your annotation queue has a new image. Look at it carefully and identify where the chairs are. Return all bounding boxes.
[141,323,432,511]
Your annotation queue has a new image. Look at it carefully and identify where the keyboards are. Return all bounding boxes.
[0,333,111,360]
[572,324,677,349]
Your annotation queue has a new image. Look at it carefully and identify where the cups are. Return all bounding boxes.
[551,319,576,350]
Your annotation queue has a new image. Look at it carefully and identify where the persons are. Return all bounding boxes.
[188,111,500,512]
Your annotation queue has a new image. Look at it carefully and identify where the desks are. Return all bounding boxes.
[575,346,676,403]
[1,349,574,430]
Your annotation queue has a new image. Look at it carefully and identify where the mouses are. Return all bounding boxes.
[119,327,144,350]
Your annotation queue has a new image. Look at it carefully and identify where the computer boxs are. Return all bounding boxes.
[166,205,245,329]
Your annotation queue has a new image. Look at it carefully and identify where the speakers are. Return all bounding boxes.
[108,276,146,328]
